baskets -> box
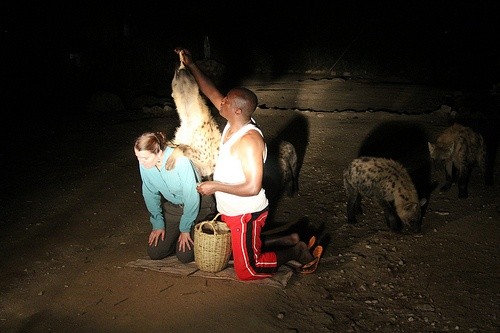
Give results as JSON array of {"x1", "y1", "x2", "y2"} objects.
[{"x1": 193, "y1": 212, "x2": 231, "y2": 272}]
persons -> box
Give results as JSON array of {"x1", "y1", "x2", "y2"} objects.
[
  {"x1": 175, "y1": 47, "x2": 323, "y2": 281},
  {"x1": 133, "y1": 131, "x2": 217, "y2": 263}
]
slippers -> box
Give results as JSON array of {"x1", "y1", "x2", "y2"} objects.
[
  {"x1": 297, "y1": 245, "x2": 323, "y2": 273},
  {"x1": 307, "y1": 236, "x2": 317, "y2": 250}
]
